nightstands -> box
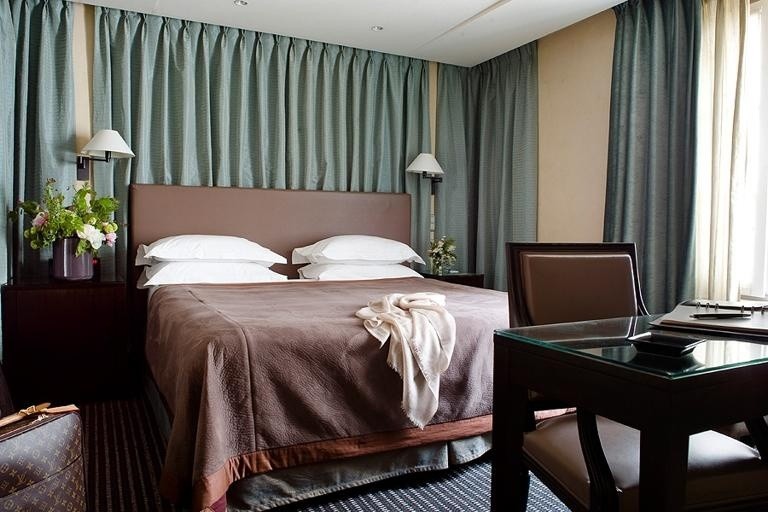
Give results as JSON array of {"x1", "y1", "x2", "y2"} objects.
[
  {"x1": 418, "y1": 270, "x2": 485, "y2": 288},
  {"x1": 0, "y1": 271, "x2": 127, "y2": 399}
]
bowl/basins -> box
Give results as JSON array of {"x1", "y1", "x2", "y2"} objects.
[
  {"x1": 622, "y1": 330, "x2": 707, "y2": 358},
  {"x1": 626, "y1": 351, "x2": 703, "y2": 377}
]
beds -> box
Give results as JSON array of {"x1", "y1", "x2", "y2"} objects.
[{"x1": 125, "y1": 181, "x2": 581, "y2": 512}]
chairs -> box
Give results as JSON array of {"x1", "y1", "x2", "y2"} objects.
[{"x1": 505, "y1": 240, "x2": 768, "y2": 511}]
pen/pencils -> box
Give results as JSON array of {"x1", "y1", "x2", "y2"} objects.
[{"x1": 690, "y1": 313, "x2": 751, "y2": 319}]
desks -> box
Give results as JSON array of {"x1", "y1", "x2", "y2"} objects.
[{"x1": 489, "y1": 311, "x2": 768, "y2": 512}]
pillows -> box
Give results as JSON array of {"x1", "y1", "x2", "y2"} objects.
[
  {"x1": 291, "y1": 233, "x2": 426, "y2": 266},
  {"x1": 295, "y1": 263, "x2": 424, "y2": 281},
  {"x1": 136, "y1": 262, "x2": 289, "y2": 290},
  {"x1": 133, "y1": 233, "x2": 289, "y2": 269}
]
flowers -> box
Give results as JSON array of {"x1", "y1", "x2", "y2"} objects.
[
  {"x1": 15, "y1": 176, "x2": 124, "y2": 255},
  {"x1": 427, "y1": 235, "x2": 458, "y2": 269}
]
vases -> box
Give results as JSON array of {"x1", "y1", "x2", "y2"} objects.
[{"x1": 52, "y1": 234, "x2": 96, "y2": 282}]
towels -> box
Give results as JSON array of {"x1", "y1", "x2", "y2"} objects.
[{"x1": 354, "y1": 291, "x2": 458, "y2": 431}]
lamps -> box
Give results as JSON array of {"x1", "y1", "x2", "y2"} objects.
[
  {"x1": 405, "y1": 152, "x2": 446, "y2": 195},
  {"x1": 75, "y1": 128, "x2": 137, "y2": 181}
]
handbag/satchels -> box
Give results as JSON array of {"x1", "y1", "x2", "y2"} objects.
[{"x1": 0, "y1": 402, "x2": 89, "y2": 512}]
